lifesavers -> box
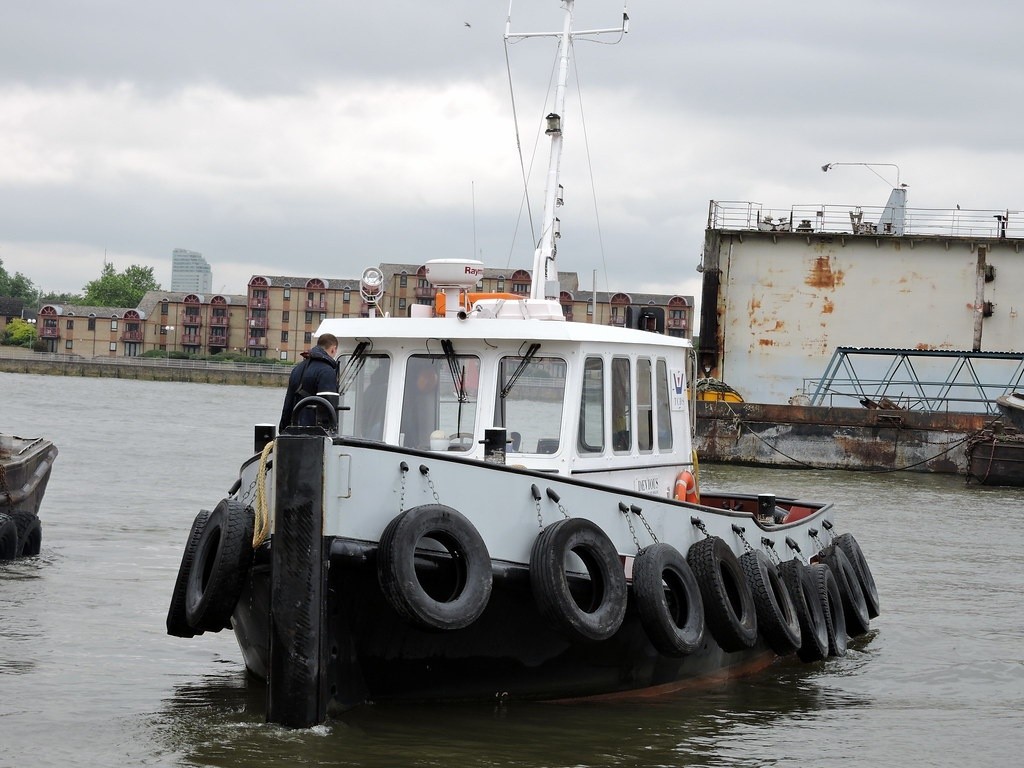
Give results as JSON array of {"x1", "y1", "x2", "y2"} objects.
[
  {"x1": 184, "y1": 498, "x2": 256, "y2": 633},
  {"x1": 166, "y1": 509, "x2": 213, "y2": 638},
  {"x1": 835, "y1": 534, "x2": 879, "y2": 619},
  {"x1": 776, "y1": 559, "x2": 829, "y2": 664},
  {"x1": 632, "y1": 545, "x2": 705, "y2": 658},
  {"x1": 11, "y1": 511, "x2": 42, "y2": 558},
  {"x1": 674, "y1": 472, "x2": 698, "y2": 505},
  {"x1": 530, "y1": 518, "x2": 628, "y2": 644},
  {"x1": 687, "y1": 536, "x2": 758, "y2": 654},
  {"x1": 0, "y1": 512, "x2": 18, "y2": 563},
  {"x1": 818, "y1": 547, "x2": 870, "y2": 638},
  {"x1": 733, "y1": 552, "x2": 803, "y2": 655},
  {"x1": 806, "y1": 563, "x2": 848, "y2": 658},
  {"x1": 376, "y1": 503, "x2": 493, "y2": 634}
]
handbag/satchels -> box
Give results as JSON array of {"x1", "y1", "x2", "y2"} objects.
[{"x1": 294, "y1": 357, "x2": 333, "y2": 398}]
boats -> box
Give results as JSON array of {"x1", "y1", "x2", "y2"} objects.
[
  {"x1": 0, "y1": 436, "x2": 59, "y2": 564},
  {"x1": 161, "y1": 1, "x2": 880, "y2": 733}
]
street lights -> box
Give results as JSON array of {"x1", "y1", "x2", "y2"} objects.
[
  {"x1": 165, "y1": 325, "x2": 175, "y2": 367},
  {"x1": 28, "y1": 318, "x2": 37, "y2": 351}
]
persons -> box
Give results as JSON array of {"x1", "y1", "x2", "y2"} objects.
[{"x1": 278, "y1": 333, "x2": 339, "y2": 437}]
